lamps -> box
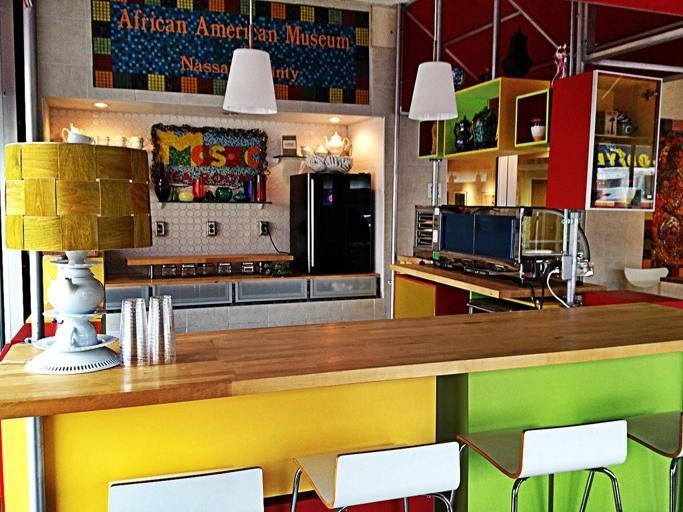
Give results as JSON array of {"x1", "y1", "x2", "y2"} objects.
[
  {"x1": 222, "y1": 0, "x2": 278, "y2": 114},
  {"x1": 4, "y1": 142, "x2": 153, "y2": 375},
  {"x1": 408, "y1": 0, "x2": 459, "y2": 121}
]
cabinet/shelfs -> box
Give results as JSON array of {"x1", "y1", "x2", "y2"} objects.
[
  {"x1": 443, "y1": 78, "x2": 552, "y2": 160},
  {"x1": 514, "y1": 88, "x2": 550, "y2": 148},
  {"x1": 546, "y1": 69, "x2": 663, "y2": 212},
  {"x1": 417, "y1": 120, "x2": 444, "y2": 160}
]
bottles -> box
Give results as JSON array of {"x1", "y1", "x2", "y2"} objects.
[{"x1": 155, "y1": 177, "x2": 232, "y2": 202}]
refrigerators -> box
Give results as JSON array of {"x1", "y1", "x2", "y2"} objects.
[{"x1": 289, "y1": 173, "x2": 374, "y2": 276}]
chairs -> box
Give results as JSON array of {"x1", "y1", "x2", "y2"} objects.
[{"x1": 624, "y1": 266, "x2": 668, "y2": 295}]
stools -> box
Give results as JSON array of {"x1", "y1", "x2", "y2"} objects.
[
  {"x1": 290, "y1": 442, "x2": 463, "y2": 512},
  {"x1": 445, "y1": 416, "x2": 628, "y2": 512},
  {"x1": 107, "y1": 463, "x2": 264, "y2": 511},
  {"x1": 580, "y1": 414, "x2": 683, "y2": 510}
]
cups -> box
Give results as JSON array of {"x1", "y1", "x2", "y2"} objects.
[
  {"x1": 62, "y1": 128, "x2": 93, "y2": 145},
  {"x1": 531, "y1": 121, "x2": 545, "y2": 142},
  {"x1": 55, "y1": 320, "x2": 100, "y2": 349},
  {"x1": 244, "y1": 174, "x2": 266, "y2": 202},
  {"x1": 119, "y1": 295, "x2": 176, "y2": 366}
]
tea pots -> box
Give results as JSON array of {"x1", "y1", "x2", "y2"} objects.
[
  {"x1": 454, "y1": 115, "x2": 474, "y2": 152},
  {"x1": 324, "y1": 130, "x2": 350, "y2": 155},
  {"x1": 50, "y1": 268, "x2": 105, "y2": 314}
]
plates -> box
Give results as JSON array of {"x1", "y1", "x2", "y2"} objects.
[
  {"x1": 43, "y1": 311, "x2": 105, "y2": 320},
  {"x1": 30, "y1": 332, "x2": 116, "y2": 350},
  {"x1": 47, "y1": 261, "x2": 100, "y2": 267}
]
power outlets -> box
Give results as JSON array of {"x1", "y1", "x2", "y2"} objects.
[
  {"x1": 260, "y1": 221, "x2": 269, "y2": 236},
  {"x1": 155, "y1": 222, "x2": 165, "y2": 235},
  {"x1": 207, "y1": 221, "x2": 216, "y2": 235}
]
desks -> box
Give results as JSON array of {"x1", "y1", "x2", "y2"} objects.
[
  {"x1": 1, "y1": 303, "x2": 682, "y2": 512},
  {"x1": 390, "y1": 264, "x2": 607, "y2": 319}
]
